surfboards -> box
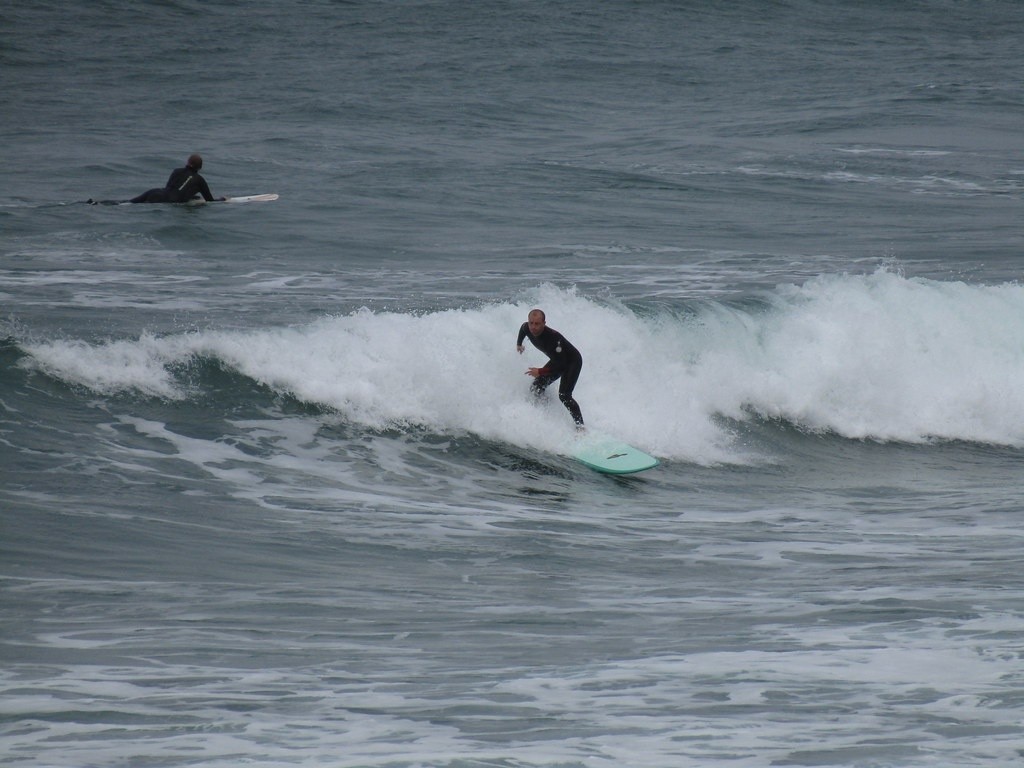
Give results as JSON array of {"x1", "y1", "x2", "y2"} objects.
[
  {"x1": 76, "y1": 192, "x2": 279, "y2": 205},
  {"x1": 554, "y1": 420, "x2": 662, "y2": 476}
]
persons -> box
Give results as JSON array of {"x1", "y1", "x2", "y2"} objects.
[
  {"x1": 517, "y1": 309, "x2": 585, "y2": 432},
  {"x1": 86, "y1": 154, "x2": 228, "y2": 205}
]
